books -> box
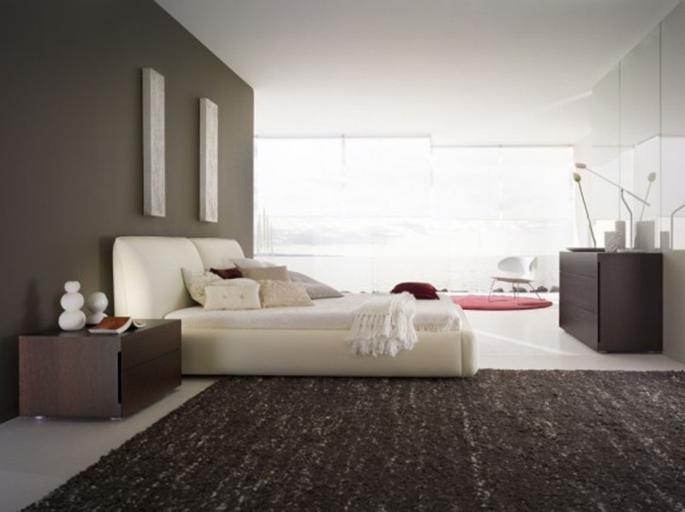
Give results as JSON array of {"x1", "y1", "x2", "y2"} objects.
[{"x1": 88, "y1": 315, "x2": 146, "y2": 335}]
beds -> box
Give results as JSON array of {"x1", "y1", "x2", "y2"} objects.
[{"x1": 110, "y1": 234, "x2": 484, "y2": 382}]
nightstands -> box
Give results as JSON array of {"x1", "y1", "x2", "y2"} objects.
[{"x1": 15, "y1": 316, "x2": 185, "y2": 429}]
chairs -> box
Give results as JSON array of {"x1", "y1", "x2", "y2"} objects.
[{"x1": 488, "y1": 252, "x2": 545, "y2": 306}]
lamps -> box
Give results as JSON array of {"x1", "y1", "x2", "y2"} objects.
[{"x1": 573, "y1": 160, "x2": 652, "y2": 250}]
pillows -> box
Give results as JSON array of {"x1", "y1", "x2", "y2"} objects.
[
  {"x1": 180, "y1": 253, "x2": 347, "y2": 312},
  {"x1": 389, "y1": 281, "x2": 440, "y2": 304}
]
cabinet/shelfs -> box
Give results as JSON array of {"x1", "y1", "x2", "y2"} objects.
[{"x1": 556, "y1": 246, "x2": 666, "y2": 360}]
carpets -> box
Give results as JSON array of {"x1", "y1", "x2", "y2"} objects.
[
  {"x1": 16, "y1": 370, "x2": 684, "y2": 510},
  {"x1": 450, "y1": 290, "x2": 556, "y2": 315}
]
